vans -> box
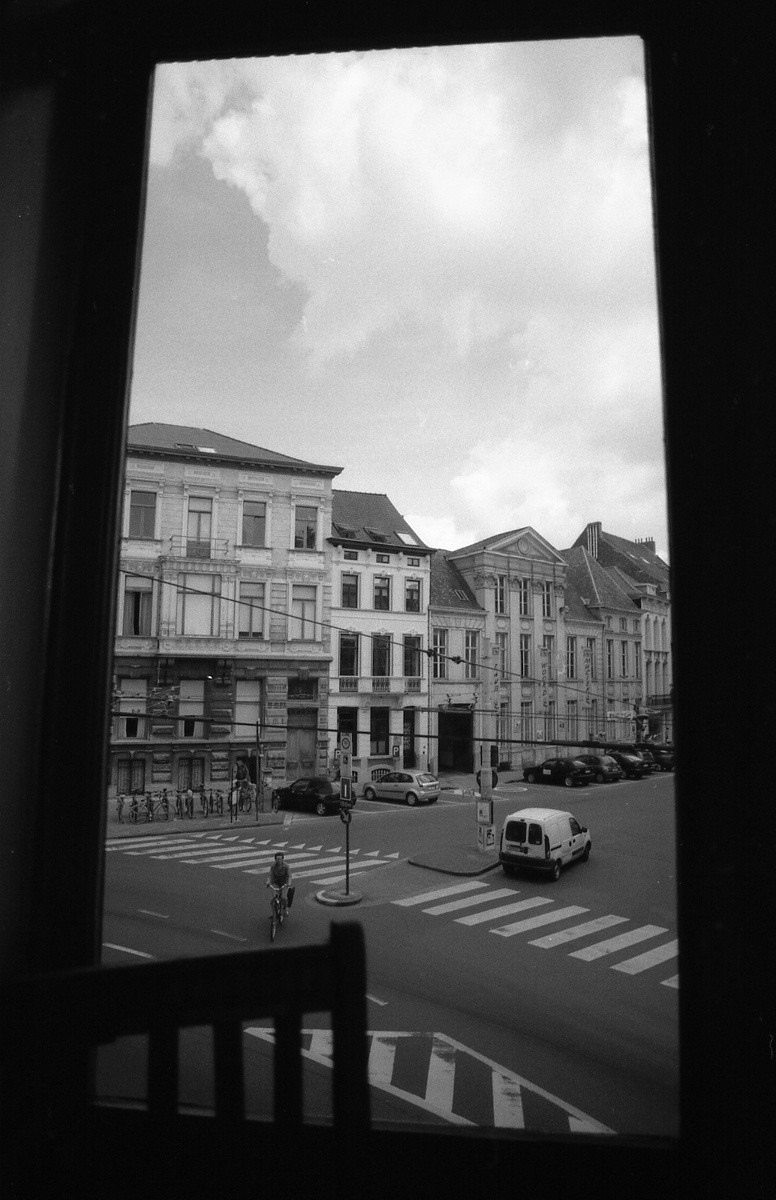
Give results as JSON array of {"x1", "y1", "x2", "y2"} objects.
[{"x1": 498, "y1": 807, "x2": 592, "y2": 883}]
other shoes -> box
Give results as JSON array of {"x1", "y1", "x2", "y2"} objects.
[
  {"x1": 269, "y1": 914, "x2": 273, "y2": 919},
  {"x1": 284, "y1": 909, "x2": 289, "y2": 917}
]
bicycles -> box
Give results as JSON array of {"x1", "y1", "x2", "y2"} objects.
[
  {"x1": 115, "y1": 777, "x2": 254, "y2": 826},
  {"x1": 263, "y1": 882, "x2": 295, "y2": 943}
]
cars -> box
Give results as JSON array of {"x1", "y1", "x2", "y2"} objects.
[
  {"x1": 605, "y1": 750, "x2": 652, "y2": 780},
  {"x1": 627, "y1": 747, "x2": 675, "y2": 773},
  {"x1": 271, "y1": 774, "x2": 357, "y2": 816},
  {"x1": 522, "y1": 757, "x2": 596, "y2": 788},
  {"x1": 574, "y1": 753, "x2": 625, "y2": 784},
  {"x1": 361, "y1": 768, "x2": 442, "y2": 806}
]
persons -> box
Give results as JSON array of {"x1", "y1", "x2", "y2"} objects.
[
  {"x1": 232, "y1": 759, "x2": 250, "y2": 805},
  {"x1": 265, "y1": 853, "x2": 292, "y2": 918}
]
